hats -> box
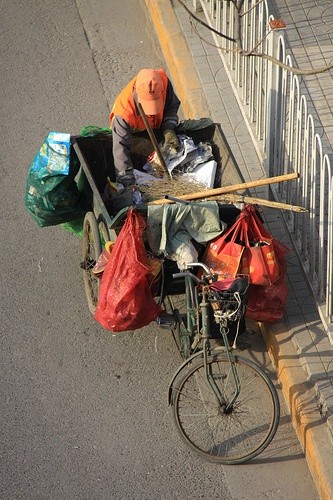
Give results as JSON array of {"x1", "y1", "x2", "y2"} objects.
[{"x1": 136, "y1": 69, "x2": 164, "y2": 115}]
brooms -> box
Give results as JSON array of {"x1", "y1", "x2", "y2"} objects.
[{"x1": 132, "y1": 164, "x2": 308, "y2": 212}]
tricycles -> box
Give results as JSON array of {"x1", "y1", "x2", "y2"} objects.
[{"x1": 70, "y1": 122, "x2": 282, "y2": 466}]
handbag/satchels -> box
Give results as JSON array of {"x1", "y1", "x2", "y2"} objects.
[{"x1": 196, "y1": 205, "x2": 283, "y2": 291}]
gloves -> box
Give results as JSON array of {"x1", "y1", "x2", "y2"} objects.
[{"x1": 163, "y1": 129, "x2": 180, "y2": 149}]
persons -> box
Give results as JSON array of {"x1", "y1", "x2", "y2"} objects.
[{"x1": 108, "y1": 68, "x2": 181, "y2": 188}]
